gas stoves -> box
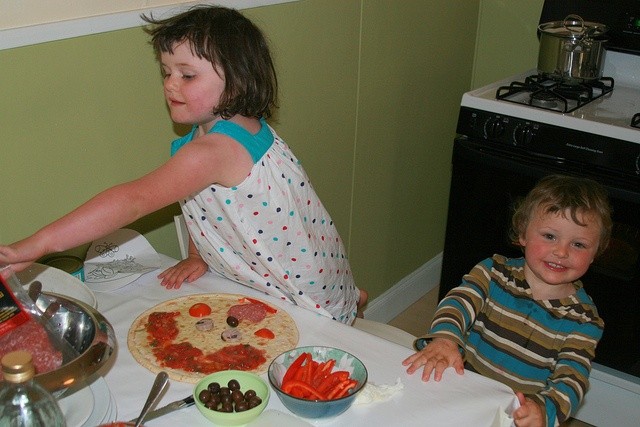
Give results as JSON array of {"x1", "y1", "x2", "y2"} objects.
[{"x1": 496, "y1": 71, "x2": 613, "y2": 114}]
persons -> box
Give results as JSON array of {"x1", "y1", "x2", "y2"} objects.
[
  {"x1": 0, "y1": 2, "x2": 360, "y2": 332},
  {"x1": 401, "y1": 171, "x2": 615, "y2": 427}
]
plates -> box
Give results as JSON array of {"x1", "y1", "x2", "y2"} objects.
[
  {"x1": 54, "y1": 374, "x2": 118, "y2": 427},
  {"x1": 16, "y1": 262, "x2": 97, "y2": 311}
]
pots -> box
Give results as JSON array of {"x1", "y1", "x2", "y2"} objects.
[{"x1": 537, "y1": 13, "x2": 610, "y2": 79}]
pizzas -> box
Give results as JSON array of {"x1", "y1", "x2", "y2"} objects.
[{"x1": 127, "y1": 292, "x2": 299, "y2": 384}]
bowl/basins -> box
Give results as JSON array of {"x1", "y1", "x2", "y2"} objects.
[
  {"x1": 268, "y1": 346, "x2": 369, "y2": 420},
  {"x1": 2, "y1": 289, "x2": 118, "y2": 405},
  {"x1": 196, "y1": 369, "x2": 269, "y2": 427}
]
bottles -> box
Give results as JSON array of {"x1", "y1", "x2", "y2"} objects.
[{"x1": 0, "y1": 350, "x2": 68, "y2": 427}]
